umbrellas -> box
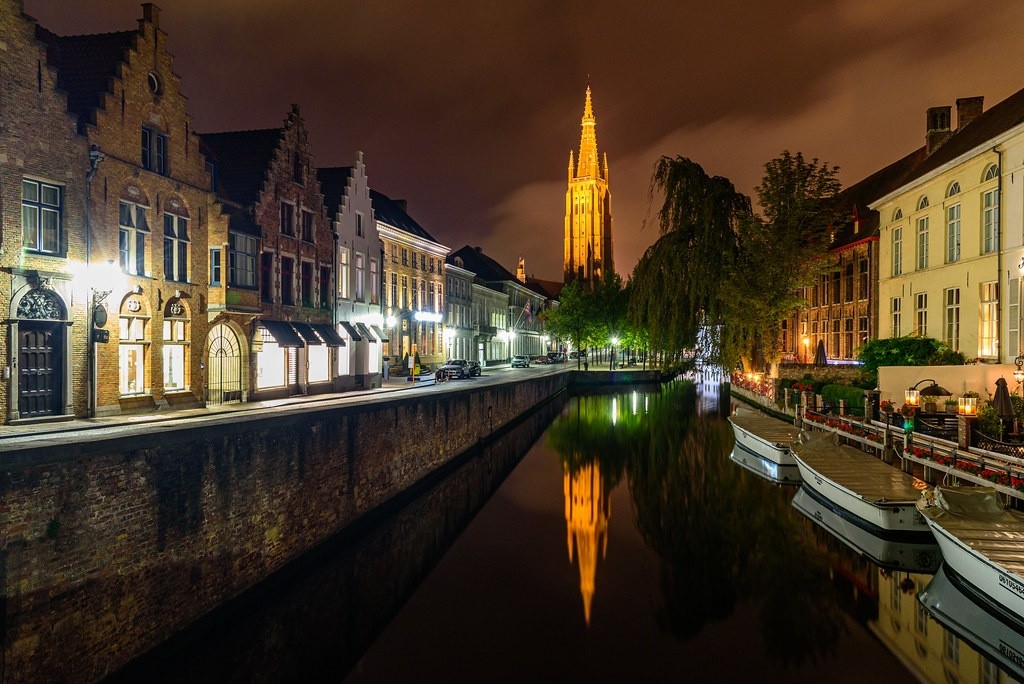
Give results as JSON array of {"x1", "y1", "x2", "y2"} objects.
[
  {"x1": 814, "y1": 339, "x2": 827, "y2": 364},
  {"x1": 993, "y1": 377, "x2": 1015, "y2": 442}
]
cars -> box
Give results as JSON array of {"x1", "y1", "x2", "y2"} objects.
[
  {"x1": 571, "y1": 352, "x2": 578, "y2": 359},
  {"x1": 535, "y1": 356, "x2": 553, "y2": 365}
]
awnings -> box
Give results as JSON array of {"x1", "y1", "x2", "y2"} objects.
[
  {"x1": 371, "y1": 325, "x2": 389, "y2": 343},
  {"x1": 259, "y1": 320, "x2": 304, "y2": 348},
  {"x1": 340, "y1": 320, "x2": 362, "y2": 342},
  {"x1": 310, "y1": 322, "x2": 346, "y2": 348},
  {"x1": 290, "y1": 321, "x2": 322, "y2": 345},
  {"x1": 356, "y1": 322, "x2": 377, "y2": 343}
]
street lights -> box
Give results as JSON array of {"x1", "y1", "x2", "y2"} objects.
[
  {"x1": 804, "y1": 338, "x2": 808, "y2": 362},
  {"x1": 613, "y1": 338, "x2": 618, "y2": 360}
]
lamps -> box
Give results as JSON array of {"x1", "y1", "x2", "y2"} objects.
[
  {"x1": 959, "y1": 394, "x2": 978, "y2": 417},
  {"x1": 1013, "y1": 356, "x2": 1024, "y2": 384},
  {"x1": 905, "y1": 386, "x2": 920, "y2": 406}
]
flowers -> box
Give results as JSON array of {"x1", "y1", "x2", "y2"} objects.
[
  {"x1": 902, "y1": 404, "x2": 915, "y2": 419},
  {"x1": 880, "y1": 399, "x2": 896, "y2": 414},
  {"x1": 904, "y1": 446, "x2": 1024, "y2": 494},
  {"x1": 804, "y1": 412, "x2": 884, "y2": 444}
]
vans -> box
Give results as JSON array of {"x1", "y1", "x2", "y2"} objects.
[{"x1": 547, "y1": 353, "x2": 564, "y2": 363}]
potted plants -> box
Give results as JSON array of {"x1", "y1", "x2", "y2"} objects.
[
  {"x1": 944, "y1": 400, "x2": 958, "y2": 414},
  {"x1": 920, "y1": 395, "x2": 940, "y2": 413}
]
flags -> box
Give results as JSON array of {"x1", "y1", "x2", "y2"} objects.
[{"x1": 523, "y1": 299, "x2": 542, "y2": 324}]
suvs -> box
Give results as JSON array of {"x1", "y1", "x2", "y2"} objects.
[
  {"x1": 442, "y1": 359, "x2": 471, "y2": 378},
  {"x1": 468, "y1": 361, "x2": 482, "y2": 376},
  {"x1": 511, "y1": 355, "x2": 530, "y2": 368}
]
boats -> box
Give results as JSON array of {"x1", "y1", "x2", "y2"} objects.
[
  {"x1": 728, "y1": 441, "x2": 801, "y2": 485},
  {"x1": 918, "y1": 560, "x2": 1024, "y2": 684},
  {"x1": 789, "y1": 429, "x2": 932, "y2": 533},
  {"x1": 727, "y1": 404, "x2": 840, "y2": 464},
  {"x1": 916, "y1": 482, "x2": 1024, "y2": 619},
  {"x1": 790, "y1": 481, "x2": 942, "y2": 575}
]
